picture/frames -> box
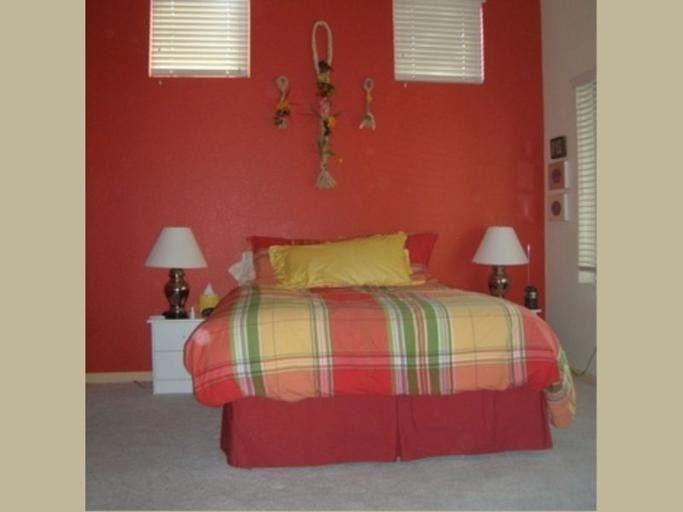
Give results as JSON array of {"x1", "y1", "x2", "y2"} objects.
[{"x1": 550, "y1": 137, "x2": 566, "y2": 159}]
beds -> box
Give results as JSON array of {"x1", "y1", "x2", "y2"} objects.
[{"x1": 181, "y1": 233, "x2": 578, "y2": 468}]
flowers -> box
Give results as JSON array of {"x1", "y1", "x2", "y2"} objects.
[
  {"x1": 275, "y1": 99, "x2": 289, "y2": 114},
  {"x1": 323, "y1": 112, "x2": 336, "y2": 135}
]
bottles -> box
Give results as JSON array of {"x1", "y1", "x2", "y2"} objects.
[{"x1": 525, "y1": 286, "x2": 538, "y2": 310}]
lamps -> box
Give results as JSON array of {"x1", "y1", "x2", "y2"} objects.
[
  {"x1": 471, "y1": 226, "x2": 530, "y2": 299},
  {"x1": 144, "y1": 227, "x2": 208, "y2": 319}
]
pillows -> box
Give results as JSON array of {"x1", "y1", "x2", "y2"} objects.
[
  {"x1": 228, "y1": 249, "x2": 255, "y2": 288},
  {"x1": 242, "y1": 232, "x2": 440, "y2": 291},
  {"x1": 268, "y1": 230, "x2": 414, "y2": 291}
]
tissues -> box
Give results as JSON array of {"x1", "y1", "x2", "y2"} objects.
[{"x1": 199, "y1": 282, "x2": 219, "y2": 310}]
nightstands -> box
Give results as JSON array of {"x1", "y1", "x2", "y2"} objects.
[
  {"x1": 527, "y1": 307, "x2": 542, "y2": 317},
  {"x1": 147, "y1": 314, "x2": 207, "y2": 395}
]
